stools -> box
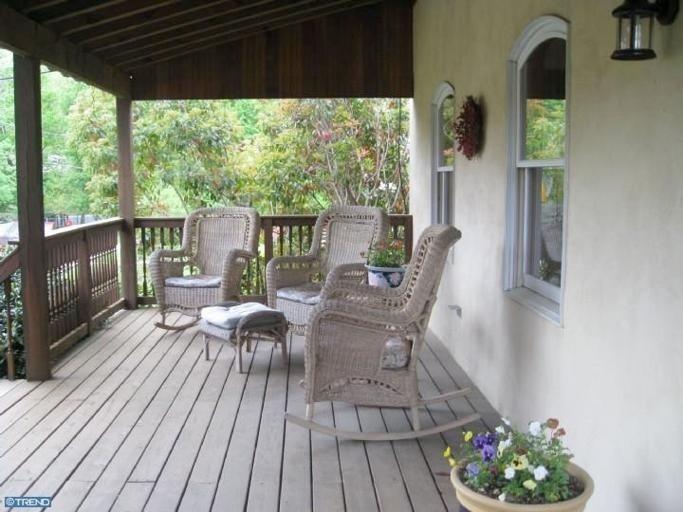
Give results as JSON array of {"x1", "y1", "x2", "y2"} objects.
[{"x1": 201, "y1": 304, "x2": 288, "y2": 374}]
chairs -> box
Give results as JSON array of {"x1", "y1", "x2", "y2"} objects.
[
  {"x1": 265, "y1": 204, "x2": 389, "y2": 349},
  {"x1": 150, "y1": 207, "x2": 261, "y2": 331},
  {"x1": 282, "y1": 224, "x2": 480, "y2": 442}
]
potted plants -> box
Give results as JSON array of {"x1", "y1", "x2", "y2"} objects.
[{"x1": 360, "y1": 238, "x2": 406, "y2": 288}]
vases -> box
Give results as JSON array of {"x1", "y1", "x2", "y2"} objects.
[{"x1": 449, "y1": 452, "x2": 594, "y2": 512}]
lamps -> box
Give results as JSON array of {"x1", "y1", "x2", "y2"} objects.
[{"x1": 610, "y1": 1, "x2": 683, "y2": 60}]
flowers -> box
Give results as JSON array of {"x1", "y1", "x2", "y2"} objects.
[{"x1": 441, "y1": 417, "x2": 573, "y2": 502}]
